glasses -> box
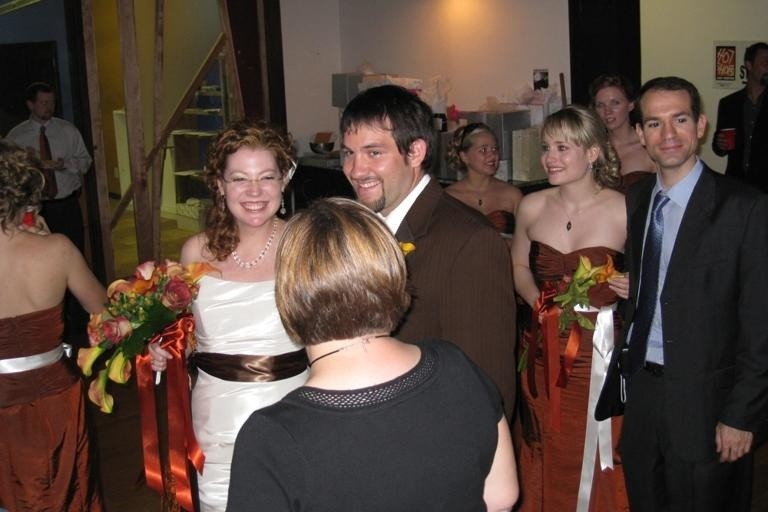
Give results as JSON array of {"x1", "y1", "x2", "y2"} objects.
[{"x1": 221, "y1": 172, "x2": 283, "y2": 187}]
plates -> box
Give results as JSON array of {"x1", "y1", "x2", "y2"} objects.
[{"x1": 40, "y1": 167, "x2": 54, "y2": 169}]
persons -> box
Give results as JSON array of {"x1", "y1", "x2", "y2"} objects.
[
  {"x1": 149, "y1": 120, "x2": 313, "y2": 512},
  {"x1": 0, "y1": 137, "x2": 109, "y2": 512},
  {"x1": 712, "y1": 43, "x2": 766, "y2": 193},
  {"x1": 341, "y1": 85, "x2": 517, "y2": 428},
  {"x1": 595, "y1": 76, "x2": 768, "y2": 512},
  {"x1": 226, "y1": 197, "x2": 521, "y2": 512},
  {"x1": 511, "y1": 105, "x2": 630, "y2": 512},
  {"x1": 5, "y1": 82, "x2": 93, "y2": 328}
]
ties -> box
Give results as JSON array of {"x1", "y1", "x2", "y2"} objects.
[
  {"x1": 627, "y1": 190, "x2": 670, "y2": 381},
  {"x1": 40, "y1": 126, "x2": 57, "y2": 198}
]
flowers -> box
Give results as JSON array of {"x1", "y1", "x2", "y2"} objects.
[
  {"x1": 76, "y1": 258, "x2": 222, "y2": 413},
  {"x1": 555, "y1": 250, "x2": 629, "y2": 346}
]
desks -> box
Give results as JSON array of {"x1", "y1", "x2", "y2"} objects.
[{"x1": 292, "y1": 146, "x2": 550, "y2": 215}]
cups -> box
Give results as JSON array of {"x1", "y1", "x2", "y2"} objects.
[
  {"x1": 721, "y1": 129, "x2": 735, "y2": 149},
  {"x1": 41, "y1": 160, "x2": 56, "y2": 167},
  {"x1": 23, "y1": 205, "x2": 34, "y2": 226}
]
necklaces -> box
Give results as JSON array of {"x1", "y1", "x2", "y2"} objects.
[{"x1": 309, "y1": 335, "x2": 393, "y2": 366}]
